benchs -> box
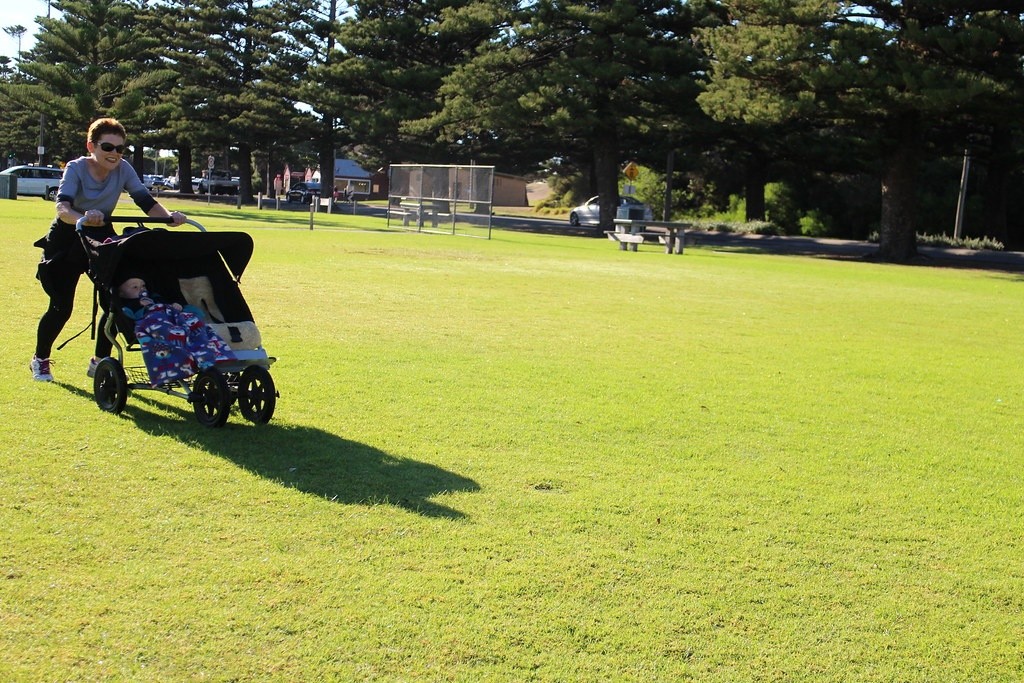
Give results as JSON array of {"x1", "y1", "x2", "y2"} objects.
[
  {"x1": 387, "y1": 210, "x2": 412, "y2": 217},
  {"x1": 629, "y1": 231, "x2": 688, "y2": 239},
  {"x1": 427, "y1": 213, "x2": 453, "y2": 218},
  {"x1": 603, "y1": 230, "x2": 665, "y2": 236}
]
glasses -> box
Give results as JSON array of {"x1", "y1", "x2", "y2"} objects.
[{"x1": 96, "y1": 140, "x2": 127, "y2": 154}]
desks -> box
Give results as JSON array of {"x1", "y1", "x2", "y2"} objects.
[
  {"x1": 399, "y1": 202, "x2": 441, "y2": 228},
  {"x1": 608, "y1": 218, "x2": 693, "y2": 255}
]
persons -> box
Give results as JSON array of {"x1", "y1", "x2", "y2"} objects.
[
  {"x1": 111, "y1": 265, "x2": 203, "y2": 352},
  {"x1": 30, "y1": 118, "x2": 187, "y2": 382}
]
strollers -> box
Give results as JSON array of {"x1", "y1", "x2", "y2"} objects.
[{"x1": 74, "y1": 212, "x2": 281, "y2": 428}]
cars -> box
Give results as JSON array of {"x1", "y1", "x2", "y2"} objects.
[
  {"x1": 285, "y1": 180, "x2": 329, "y2": 205},
  {"x1": 570, "y1": 194, "x2": 654, "y2": 229},
  {"x1": 143, "y1": 175, "x2": 242, "y2": 190}
]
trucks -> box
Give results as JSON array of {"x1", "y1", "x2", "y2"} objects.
[{"x1": 198, "y1": 169, "x2": 239, "y2": 196}]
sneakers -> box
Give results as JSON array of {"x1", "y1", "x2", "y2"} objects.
[
  {"x1": 30, "y1": 353, "x2": 54, "y2": 382},
  {"x1": 87, "y1": 356, "x2": 130, "y2": 381}
]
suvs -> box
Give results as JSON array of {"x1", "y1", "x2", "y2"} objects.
[{"x1": 0, "y1": 165, "x2": 64, "y2": 202}]
